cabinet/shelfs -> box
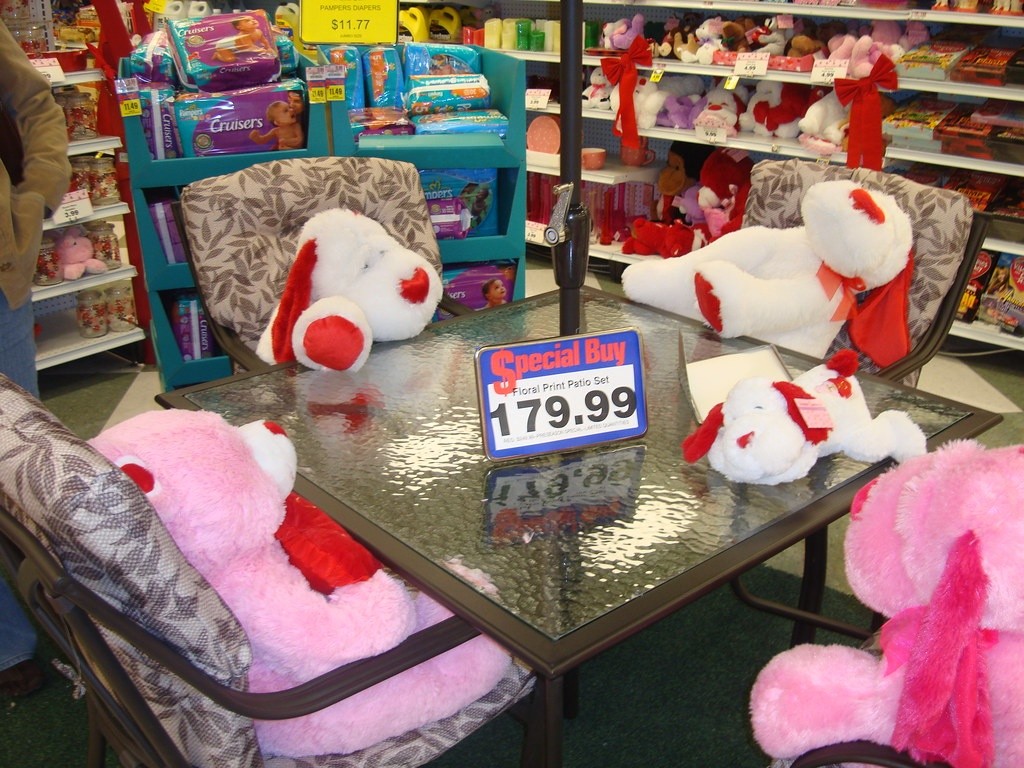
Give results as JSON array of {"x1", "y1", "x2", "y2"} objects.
[{"x1": 0, "y1": 0, "x2": 1024, "y2": 373}]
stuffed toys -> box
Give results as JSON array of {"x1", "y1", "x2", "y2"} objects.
[
  {"x1": 80, "y1": 409, "x2": 511, "y2": 756},
  {"x1": 581, "y1": 68, "x2": 896, "y2": 154},
  {"x1": 256, "y1": 207, "x2": 445, "y2": 375},
  {"x1": 749, "y1": 437, "x2": 1023, "y2": 768},
  {"x1": 620, "y1": 180, "x2": 914, "y2": 367},
  {"x1": 623, "y1": 140, "x2": 753, "y2": 260},
  {"x1": 681, "y1": 352, "x2": 927, "y2": 484},
  {"x1": 598, "y1": 12, "x2": 929, "y2": 79},
  {"x1": 45, "y1": 227, "x2": 107, "y2": 279},
  {"x1": 475, "y1": 2, "x2": 501, "y2": 21}
]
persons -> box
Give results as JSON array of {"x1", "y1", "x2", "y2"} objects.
[
  {"x1": 249, "y1": 91, "x2": 307, "y2": 150},
  {"x1": 482, "y1": 279, "x2": 507, "y2": 308},
  {"x1": 211, "y1": 17, "x2": 273, "y2": 62},
  {"x1": 0, "y1": 17, "x2": 72, "y2": 699},
  {"x1": 461, "y1": 183, "x2": 488, "y2": 223}
]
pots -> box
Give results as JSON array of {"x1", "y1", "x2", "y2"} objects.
[{"x1": 42, "y1": 46, "x2": 89, "y2": 72}]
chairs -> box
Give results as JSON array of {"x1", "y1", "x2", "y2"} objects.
[
  {"x1": 731, "y1": 158, "x2": 993, "y2": 647},
  {"x1": 0, "y1": 373, "x2": 542, "y2": 768},
  {"x1": 171, "y1": 156, "x2": 474, "y2": 372}
]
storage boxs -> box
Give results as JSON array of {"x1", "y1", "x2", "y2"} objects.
[
  {"x1": 895, "y1": 27, "x2": 991, "y2": 81},
  {"x1": 949, "y1": 36, "x2": 1024, "y2": 86},
  {"x1": 882, "y1": 95, "x2": 959, "y2": 138},
  {"x1": 933, "y1": 104, "x2": 999, "y2": 146}
]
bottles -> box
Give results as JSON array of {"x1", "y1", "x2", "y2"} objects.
[{"x1": 2, "y1": 8, "x2": 137, "y2": 338}]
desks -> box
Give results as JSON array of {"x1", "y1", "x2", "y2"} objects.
[{"x1": 153, "y1": 286, "x2": 1005, "y2": 768}]
